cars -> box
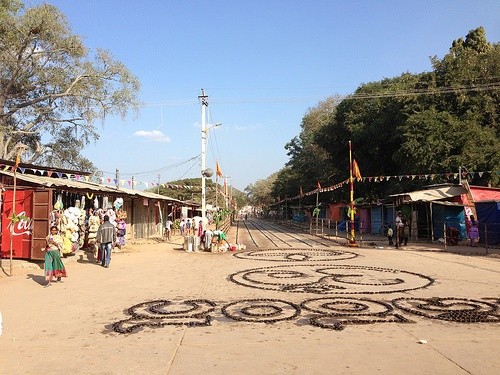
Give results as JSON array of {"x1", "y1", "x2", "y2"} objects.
[{"x1": 207, "y1": 217, "x2": 212, "y2": 225}]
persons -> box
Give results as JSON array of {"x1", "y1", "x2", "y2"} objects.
[
  {"x1": 97, "y1": 219, "x2": 107, "y2": 265},
  {"x1": 205, "y1": 230, "x2": 213, "y2": 249},
  {"x1": 200, "y1": 230, "x2": 207, "y2": 249},
  {"x1": 41, "y1": 226, "x2": 67, "y2": 288},
  {"x1": 179, "y1": 219, "x2": 186, "y2": 235},
  {"x1": 235, "y1": 206, "x2": 281, "y2": 222},
  {"x1": 198, "y1": 220, "x2": 204, "y2": 237},
  {"x1": 387, "y1": 210, "x2": 411, "y2": 248},
  {"x1": 192, "y1": 219, "x2": 196, "y2": 235},
  {"x1": 165, "y1": 218, "x2": 173, "y2": 239},
  {"x1": 97, "y1": 215, "x2": 116, "y2": 268},
  {"x1": 78, "y1": 207, "x2": 123, "y2": 253},
  {"x1": 305, "y1": 207, "x2": 312, "y2": 226},
  {"x1": 467, "y1": 215, "x2": 479, "y2": 246},
  {"x1": 186, "y1": 219, "x2": 191, "y2": 236},
  {"x1": 212, "y1": 229, "x2": 226, "y2": 244}
]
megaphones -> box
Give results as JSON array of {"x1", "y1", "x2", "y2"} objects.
[{"x1": 201, "y1": 168, "x2": 213, "y2": 177}]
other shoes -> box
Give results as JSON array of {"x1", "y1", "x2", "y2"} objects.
[
  {"x1": 404, "y1": 243, "x2": 407, "y2": 246},
  {"x1": 55, "y1": 277, "x2": 61, "y2": 282},
  {"x1": 389, "y1": 242, "x2": 392, "y2": 245},
  {"x1": 45, "y1": 283, "x2": 52, "y2": 287},
  {"x1": 100, "y1": 263, "x2": 108, "y2": 268}
]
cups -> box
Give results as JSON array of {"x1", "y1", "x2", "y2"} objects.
[{"x1": 49, "y1": 236, "x2": 53, "y2": 241}]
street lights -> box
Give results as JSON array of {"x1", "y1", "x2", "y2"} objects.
[{"x1": 201, "y1": 122, "x2": 223, "y2": 229}]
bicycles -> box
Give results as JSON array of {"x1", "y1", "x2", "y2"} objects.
[{"x1": 164, "y1": 229, "x2": 171, "y2": 241}]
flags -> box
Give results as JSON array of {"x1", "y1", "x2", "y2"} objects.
[
  {"x1": 216, "y1": 161, "x2": 222, "y2": 178},
  {"x1": 352, "y1": 160, "x2": 362, "y2": 182},
  {"x1": 318, "y1": 182, "x2": 322, "y2": 189}
]
